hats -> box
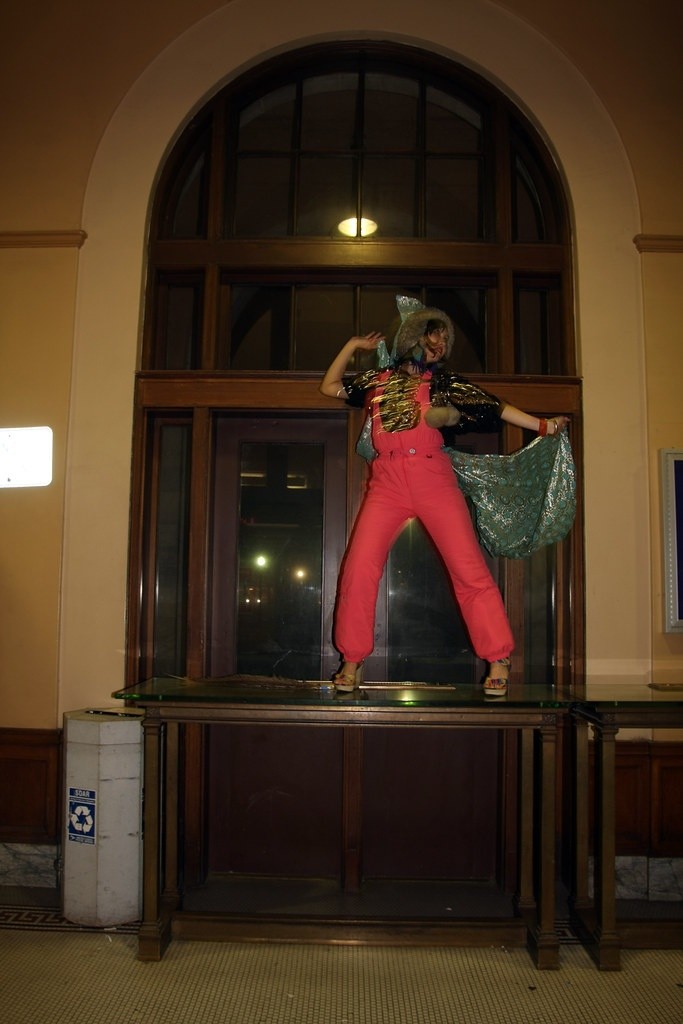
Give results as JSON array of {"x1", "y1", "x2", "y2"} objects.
[{"x1": 397, "y1": 307, "x2": 454, "y2": 362}]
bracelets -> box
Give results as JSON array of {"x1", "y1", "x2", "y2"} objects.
[
  {"x1": 537, "y1": 417, "x2": 548, "y2": 437},
  {"x1": 550, "y1": 417, "x2": 558, "y2": 436}
]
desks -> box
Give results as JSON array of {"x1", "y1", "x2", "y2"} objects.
[{"x1": 107, "y1": 678, "x2": 683, "y2": 972}]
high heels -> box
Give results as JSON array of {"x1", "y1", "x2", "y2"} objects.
[
  {"x1": 483, "y1": 658, "x2": 512, "y2": 695},
  {"x1": 332, "y1": 662, "x2": 363, "y2": 691}
]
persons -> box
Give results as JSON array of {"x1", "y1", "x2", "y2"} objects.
[{"x1": 318, "y1": 294, "x2": 570, "y2": 699}]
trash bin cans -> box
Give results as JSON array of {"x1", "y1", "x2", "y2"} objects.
[{"x1": 58, "y1": 706, "x2": 144, "y2": 927}]
